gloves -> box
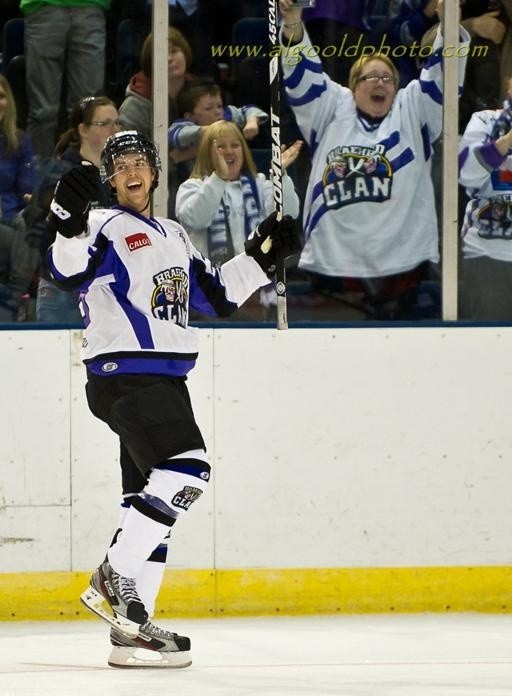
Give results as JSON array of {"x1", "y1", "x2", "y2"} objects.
[
  {"x1": 47, "y1": 165, "x2": 105, "y2": 239},
  {"x1": 244, "y1": 210, "x2": 301, "y2": 278}
]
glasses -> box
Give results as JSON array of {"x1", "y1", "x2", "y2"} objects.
[
  {"x1": 357, "y1": 72, "x2": 396, "y2": 84},
  {"x1": 87, "y1": 120, "x2": 121, "y2": 132}
]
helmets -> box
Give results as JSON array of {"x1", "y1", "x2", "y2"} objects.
[{"x1": 100, "y1": 128, "x2": 162, "y2": 193}]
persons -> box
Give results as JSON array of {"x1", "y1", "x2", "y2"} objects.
[
  {"x1": 1, "y1": 0, "x2": 509, "y2": 322},
  {"x1": 39, "y1": 129, "x2": 303, "y2": 658}
]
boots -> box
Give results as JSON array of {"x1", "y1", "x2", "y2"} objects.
[{"x1": 90, "y1": 553, "x2": 190, "y2": 651}]
